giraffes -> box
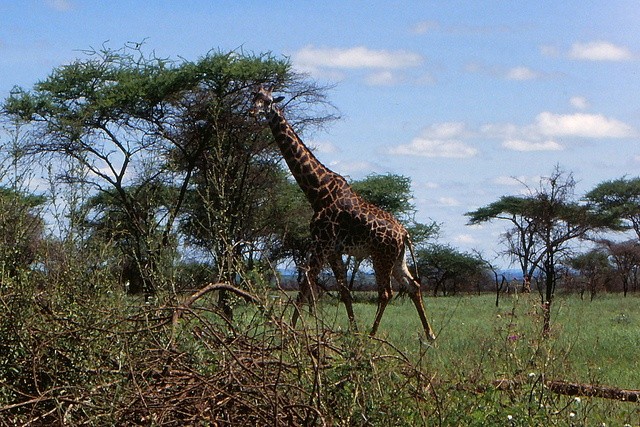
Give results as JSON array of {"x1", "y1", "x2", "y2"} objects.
[{"x1": 247, "y1": 82, "x2": 437, "y2": 343}]
